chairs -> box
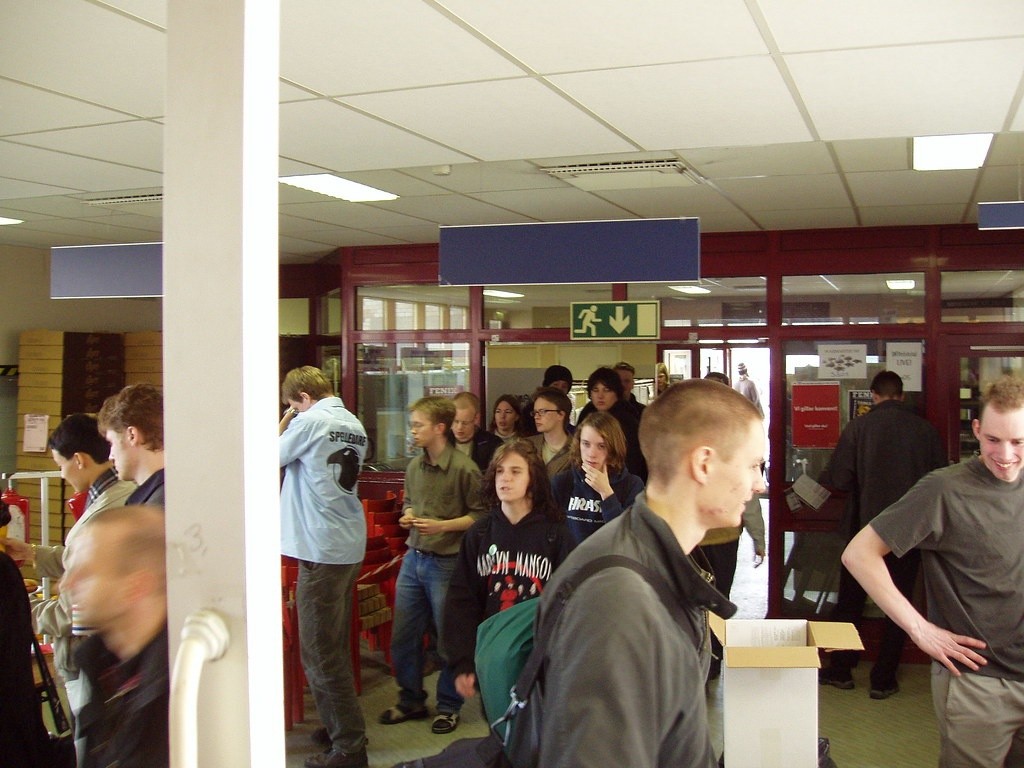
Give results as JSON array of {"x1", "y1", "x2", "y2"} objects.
[{"x1": 279, "y1": 488, "x2": 429, "y2": 732}]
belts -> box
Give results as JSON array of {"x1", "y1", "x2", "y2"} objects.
[{"x1": 415, "y1": 548, "x2": 456, "y2": 558}]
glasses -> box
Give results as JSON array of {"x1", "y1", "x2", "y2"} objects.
[{"x1": 530, "y1": 408, "x2": 560, "y2": 417}]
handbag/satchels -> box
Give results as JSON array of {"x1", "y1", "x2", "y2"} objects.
[
  {"x1": 391, "y1": 736, "x2": 511, "y2": 768},
  {"x1": 474, "y1": 597, "x2": 542, "y2": 760}
]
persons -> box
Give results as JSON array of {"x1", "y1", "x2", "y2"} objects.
[
  {"x1": 281, "y1": 366, "x2": 372, "y2": 767},
  {"x1": 506, "y1": 378, "x2": 770, "y2": 768},
  {"x1": 376, "y1": 361, "x2": 765, "y2": 736},
  {"x1": 840, "y1": 373, "x2": 1024, "y2": 768},
  {"x1": 819, "y1": 371, "x2": 950, "y2": 699},
  {"x1": 0, "y1": 382, "x2": 172, "y2": 768}
]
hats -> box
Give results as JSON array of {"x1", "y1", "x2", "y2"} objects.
[
  {"x1": 737, "y1": 363, "x2": 747, "y2": 374},
  {"x1": 542, "y1": 365, "x2": 572, "y2": 394},
  {"x1": 613, "y1": 362, "x2": 635, "y2": 375}
]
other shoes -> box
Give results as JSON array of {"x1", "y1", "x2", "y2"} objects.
[
  {"x1": 819, "y1": 668, "x2": 855, "y2": 689},
  {"x1": 870, "y1": 680, "x2": 899, "y2": 699},
  {"x1": 303, "y1": 749, "x2": 367, "y2": 768},
  {"x1": 379, "y1": 703, "x2": 428, "y2": 724},
  {"x1": 432, "y1": 712, "x2": 459, "y2": 733},
  {"x1": 310, "y1": 727, "x2": 333, "y2": 745}
]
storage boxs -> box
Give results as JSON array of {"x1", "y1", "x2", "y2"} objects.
[{"x1": 705, "y1": 611, "x2": 866, "y2": 768}]
